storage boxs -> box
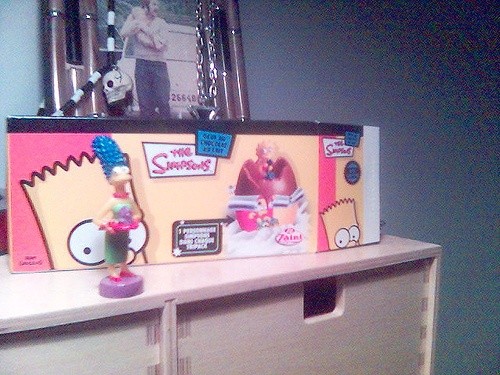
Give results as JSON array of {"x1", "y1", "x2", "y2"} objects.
[{"x1": 4, "y1": 113, "x2": 385, "y2": 276}]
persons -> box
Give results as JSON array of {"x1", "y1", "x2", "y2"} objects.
[
  {"x1": 119, "y1": 0, "x2": 173, "y2": 120},
  {"x1": 132, "y1": 6, "x2": 167, "y2": 50}
]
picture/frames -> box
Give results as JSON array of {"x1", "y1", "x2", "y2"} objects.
[{"x1": 45, "y1": 2, "x2": 251, "y2": 124}]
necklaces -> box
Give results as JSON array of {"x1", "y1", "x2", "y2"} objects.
[{"x1": 187, "y1": 0, "x2": 222, "y2": 120}]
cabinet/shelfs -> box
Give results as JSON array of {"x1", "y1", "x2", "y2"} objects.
[{"x1": 2, "y1": 229, "x2": 441, "y2": 374}]
